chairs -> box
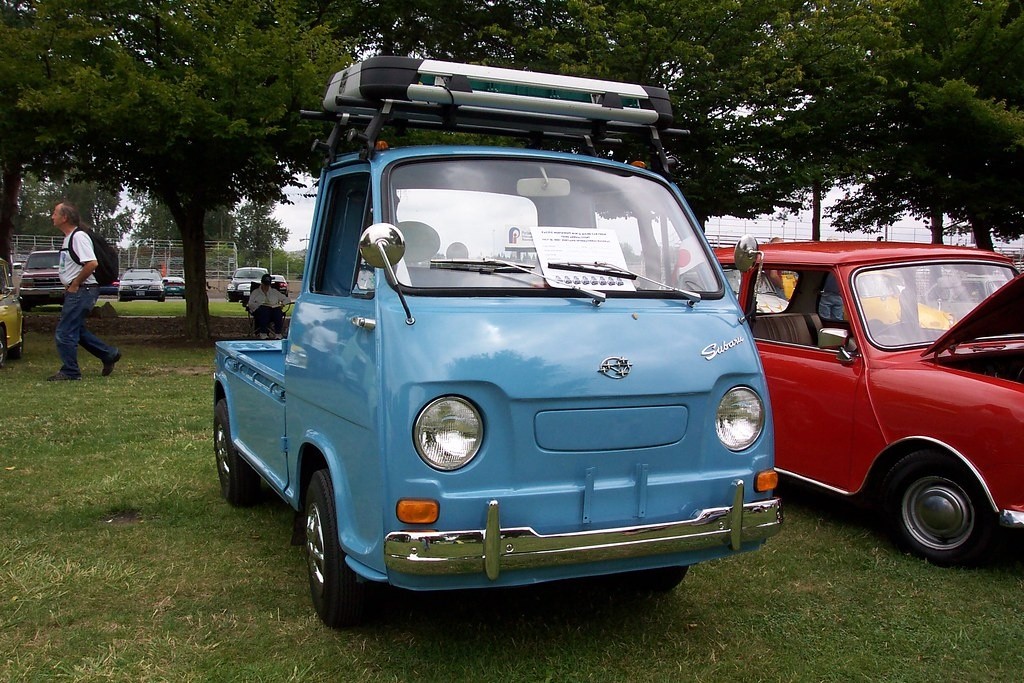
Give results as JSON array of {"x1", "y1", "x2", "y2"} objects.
[
  {"x1": 240, "y1": 282, "x2": 295, "y2": 340},
  {"x1": 394, "y1": 220, "x2": 441, "y2": 267}
]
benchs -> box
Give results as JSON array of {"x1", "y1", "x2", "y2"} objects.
[{"x1": 754, "y1": 312, "x2": 822, "y2": 346}]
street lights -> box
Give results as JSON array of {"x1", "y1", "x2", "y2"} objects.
[{"x1": 299, "y1": 239, "x2": 309, "y2": 250}]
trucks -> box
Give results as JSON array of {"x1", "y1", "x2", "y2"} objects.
[{"x1": 211, "y1": 56, "x2": 780, "y2": 629}]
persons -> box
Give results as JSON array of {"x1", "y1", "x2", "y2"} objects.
[
  {"x1": 818, "y1": 235, "x2": 844, "y2": 320},
  {"x1": 47, "y1": 201, "x2": 121, "y2": 380},
  {"x1": 248, "y1": 274, "x2": 291, "y2": 340},
  {"x1": 764, "y1": 238, "x2": 789, "y2": 301}
]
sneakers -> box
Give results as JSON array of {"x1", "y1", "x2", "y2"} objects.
[
  {"x1": 101, "y1": 348, "x2": 122, "y2": 376},
  {"x1": 46, "y1": 372, "x2": 82, "y2": 382},
  {"x1": 259, "y1": 333, "x2": 269, "y2": 340},
  {"x1": 274, "y1": 334, "x2": 283, "y2": 340}
]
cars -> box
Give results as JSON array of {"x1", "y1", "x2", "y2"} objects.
[
  {"x1": 12, "y1": 249, "x2": 66, "y2": 312},
  {"x1": 920, "y1": 273, "x2": 1010, "y2": 320},
  {"x1": 163, "y1": 277, "x2": 186, "y2": 299},
  {"x1": 226, "y1": 267, "x2": 270, "y2": 302},
  {"x1": 779, "y1": 270, "x2": 958, "y2": 333},
  {"x1": 663, "y1": 240, "x2": 1024, "y2": 569},
  {"x1": 0, "y1": 259, "x2": 24, "y2": 365},
  {"x1": 99, "y1": 279, "x2": 119, "y2": 295},
  {"x1": 118, "y1": 267, "x2": 169, "y2": 302},
  {"x1": 270, "y1": 275, "x2": 290, "y2": 297}
]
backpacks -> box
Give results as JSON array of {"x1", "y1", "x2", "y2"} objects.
[{"x1": 68, "y1": 227, "x2": 119, "y2": 287}]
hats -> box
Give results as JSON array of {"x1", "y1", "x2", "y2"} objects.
[{"x1": 262, "y1": 274, "x2": 272, "y2": 285}]
